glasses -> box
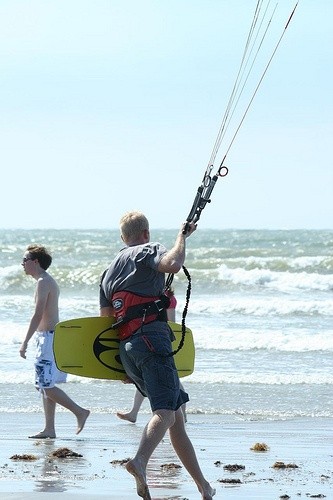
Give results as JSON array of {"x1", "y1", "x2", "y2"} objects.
[{"x1": 23, "y1": 258, "x2": 34, "y2": 263}]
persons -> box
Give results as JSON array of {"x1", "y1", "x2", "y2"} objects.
[
  {"x1": 19, "y1": 243, "x2": 90, "y2": 438},
  {"x1": 99, "y1": 211, "x2": 216, "y2": 500},
  {"x1": 117, "y1": 284, "x2": 187, "y2": 423}
]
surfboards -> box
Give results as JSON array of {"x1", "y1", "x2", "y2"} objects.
[{"x1": 52, "y1": 316, "x2": 195, "y2": 385}]
